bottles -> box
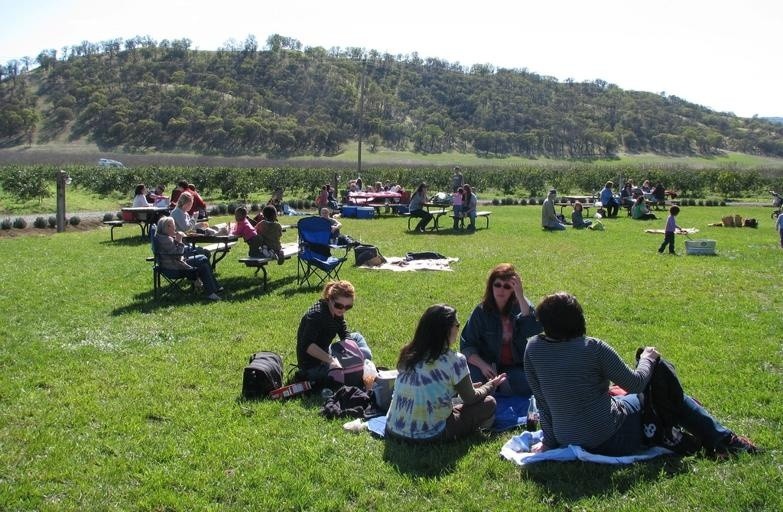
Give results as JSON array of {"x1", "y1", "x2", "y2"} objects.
[
  {"x1": 527, "y1": 395, "x2": 539, "y2": 432},
  {"x1": 488, "y1": 363, "x2": 498, "y2": 395}
]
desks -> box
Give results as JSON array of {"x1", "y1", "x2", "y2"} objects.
[
  {"x1": 123, "y1": 207, "x2": 171, "y2": 240},
  {"x1": 423, "y1": 202, "x2": 452, "y2": 229},
  {"x1": 183, "y1": 223, "x2": 291, "y2": 269},
  {"x1": 564, "y1": 195, "x2": 595, "y2": 217}
]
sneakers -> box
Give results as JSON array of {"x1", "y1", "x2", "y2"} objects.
[
  {"x1": 269, "y1": 250, "x2": 284, "y2": 265},
  {"x1": 210, "y1": 293, "x2": 223, "y2": 301},
  {"x1": 718, "y1": 434, "x2": 756, "y2": 462}
]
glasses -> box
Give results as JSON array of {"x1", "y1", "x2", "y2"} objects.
[
  {"x1": 493, "y1": 283, "x2": 512, "y2": 289},
  {"x1": 452, "y1": 321, "x2": 461, "y2": 328},
  {"x1": 333, "y1": 299, "x2": 353, "y2": 311}
]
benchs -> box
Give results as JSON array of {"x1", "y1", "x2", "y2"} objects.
[
  {"x1": 554, "y1": 203, "x2": 601, "y2": 218},
  {"x1": 238, "y1": 245, "x2": 299, "y2": 292},
  {"x1": 146, "y1": 241, "x2": 237, "y2": 271},
  {"x1": 449, "y1": 211, "x2": 493, "y2": 230},
  {"x1": 104, "y1": 220, "x2": 130, "y2": 242},
  {"x1": 406, "y1": 211, "x2": 450, "y2": 229}
]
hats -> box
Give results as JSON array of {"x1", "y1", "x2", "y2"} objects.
[{"x1": 419, "y1": 182, "x2": 430, "y2": 188}]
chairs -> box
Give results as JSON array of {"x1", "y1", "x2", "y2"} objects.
[
  {"x1": 297, "y1": 217, "x2": 347, "y2": 289},
  {"x1": 150, "y1": 223, "x2": 199, "y2": 302}
]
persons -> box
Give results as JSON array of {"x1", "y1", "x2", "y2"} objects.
[
  {"x1": 454, "y1": 187, "x2": 465, "y2": 231},
  {"x1": 461, "y1": 183, "x2": 476, "y2": 233},
  {"x1": 256, "y1": 206, "x2": 284, "y2": 266},
  {"x1": 524, "y1": 293, "x2": 764, "y2": 462},
  {"x1": 641, "y1": 180, "x2": 650, "y2": 192},
  {"x1": 460, "y1": 264, "x2": 538, "y2": 396},
  {"x1": 410, "y1": 184, "x2": 432, "y2": 233},
  {"x1": 629, "y1": 178, "x2": 637, "y2": 188},
  {"x1": 171, "y1": 192, "x2": 200, "y2": 233},
  {"x1": 571, "y1": 202, "x2": 592, "y2": 229},
  {"x1": 384, "y1": 303, "x2": 507, "y2": 445},
  {"x1": 601, "y1": 181, "x2": 619, "y2": 218},
  {"x1": 320, "y1": 208, "x2": 342, "y2": 241},
  {"x1": 296, "y1": 281, "x2": 371, "y2": 383},
  {"x1": 542, "y1": 189, "x2": 565, "y2": 231},
  {"x1": 631, "y1": 197, "x2": 654, "y2": 220},
  {"x1": 652, "y1": 181, "x2": 667, "y2": 210},
  {"x1": 156, "y1": 216, "x2": 224, "y2": 301},
  {"x1": 146, "y1": 184, "x2": 169, "y2": 206},
  {"x1": 453, "y1": 166, "x2": 464, "y2": 191},
  {"x1": 232, "y1": 207, "x2": 279, "y2": 261},
  {"x1": 622, "y1": 183, "x2": 634, "y2": 215},
  {"x1": 658, "y1": 205, "x2": 682, "y2": 255},
  {"x1": 775, "y1": 204, "x2": 783, "y2": 248},
  {"x1": 132, "y1": 184, "x2": 153, "y2": 208},
  {"x1": 171, "y1": 180, "x2": 206, "y2": 218},
  {"x1": 317, "y1": 178, "x2": 409, "y2": 217}
]
